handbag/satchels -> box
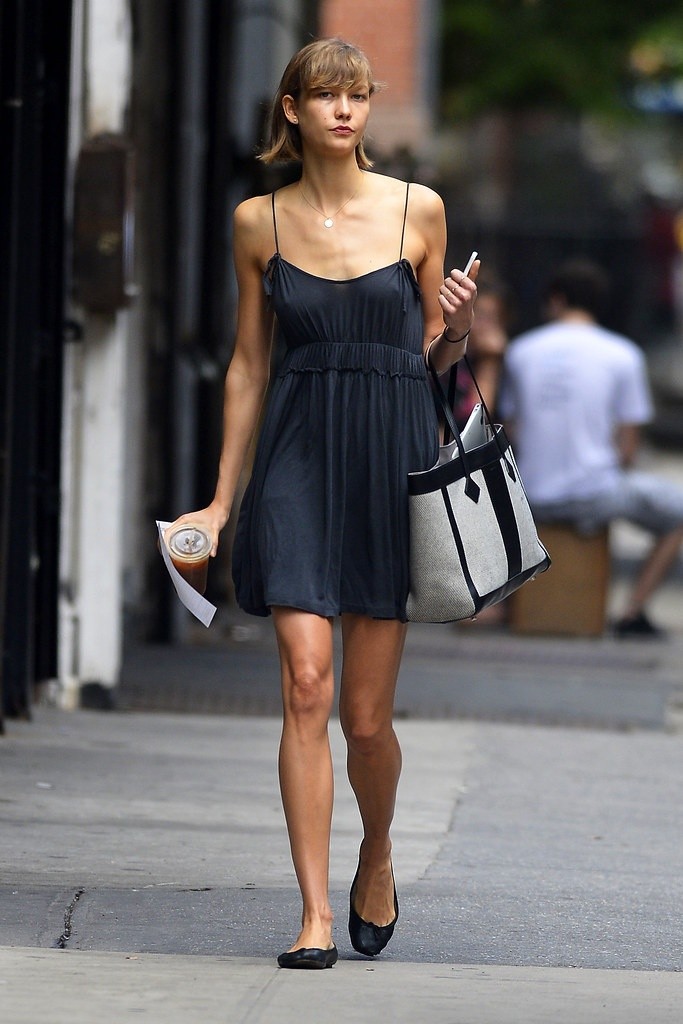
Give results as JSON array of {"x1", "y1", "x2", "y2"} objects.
[{"x1": 397, "y1": 344, "x2": 554, "y2": 623}]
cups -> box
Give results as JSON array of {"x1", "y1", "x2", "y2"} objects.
[{"x1": 167, "y1": 523, "x2": 210, "y2": 597}]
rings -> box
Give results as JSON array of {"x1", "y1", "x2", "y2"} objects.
[
  {"x1": 458, "y1": 275, "x2": 468, "y2": 286},
  {"x1": 451, "y1": 284, "x2": 461, "y2": 294}
]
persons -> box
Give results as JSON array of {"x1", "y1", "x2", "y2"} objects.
[
  {"x1": 507, "y1": 243, "x2": 683, "y2": 643},
  {"x1": 151, "y1": 32, "x2": 480, "y2": 972},
  {"x1": 437, "y1": 247, "x2": 505, "y2": 468}
]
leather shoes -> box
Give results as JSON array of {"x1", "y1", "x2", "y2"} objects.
[
  {"x1": 348, "y1": 837, "x2": 399, "y2": 958},
  {"x1": 277, "y1": 941, "x2": 338, "y2": 969}
]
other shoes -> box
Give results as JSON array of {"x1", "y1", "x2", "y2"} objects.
[{"x1": 614, "y1": 609, "x2": 670, "y2": 643}]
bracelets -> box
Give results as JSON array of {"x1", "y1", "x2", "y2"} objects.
[{"x1": 441, "y1": 322, "x2": 471, "y2": 344}]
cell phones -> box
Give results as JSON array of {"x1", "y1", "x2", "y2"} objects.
[{"x1": 465, "y1": 251, "x2": 479, "y2": 280}]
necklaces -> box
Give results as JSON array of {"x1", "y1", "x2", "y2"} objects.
[{"x1": 299, "y1": 169, "x2": 365, "y2": 229}]
